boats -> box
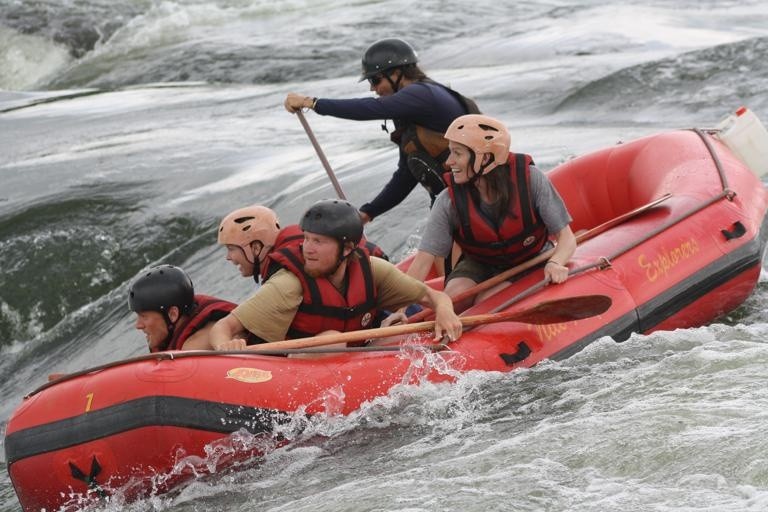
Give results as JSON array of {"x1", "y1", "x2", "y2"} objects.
[{"x1": 6, "y1": 127, "x2": 768, "y2": 512}]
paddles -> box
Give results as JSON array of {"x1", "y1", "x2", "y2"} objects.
[
  {"x1": 392, "y1": 190, "x2": 672, "y2": 326},
  {"x1": 244, "y1": 294, "x2": 613, "y2": 356}
]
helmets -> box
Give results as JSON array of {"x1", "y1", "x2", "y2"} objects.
[
  {"x1": 357, "y1": 38, "x2": 419, "y2": 92},
  {"x1": 443, "y1": 114, "x2": 511, "y2": 185},
  {"x1": 217, "y1": 205, "x2": 282, "y2": 284},
  {"x1": 127, "y1": 264, "x2": 195, "y2": 350},
  {"x1": 299, "y1": 198, "x2": 364, "y2": 274}
]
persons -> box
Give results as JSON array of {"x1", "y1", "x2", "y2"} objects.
[
  {"x1": 285, "y1": 39, "x2": 482, "y2": 225},
  {"x1": 210, "y1": 199, "x2": 462, "y2": 352},
  {"x1": 128, "y1": 264, "x2": 239, "y2": 353},
  {"x1": 379, "y1": 114, "x2": 576, "y2": 328},
  {"x1": 218, "y1": 206, "x2": 389, "y2": 285}
]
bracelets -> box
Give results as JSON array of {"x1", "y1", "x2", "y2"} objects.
[{"x1": 546, "y1": 261, "x2": 559, "y2": 266}]
